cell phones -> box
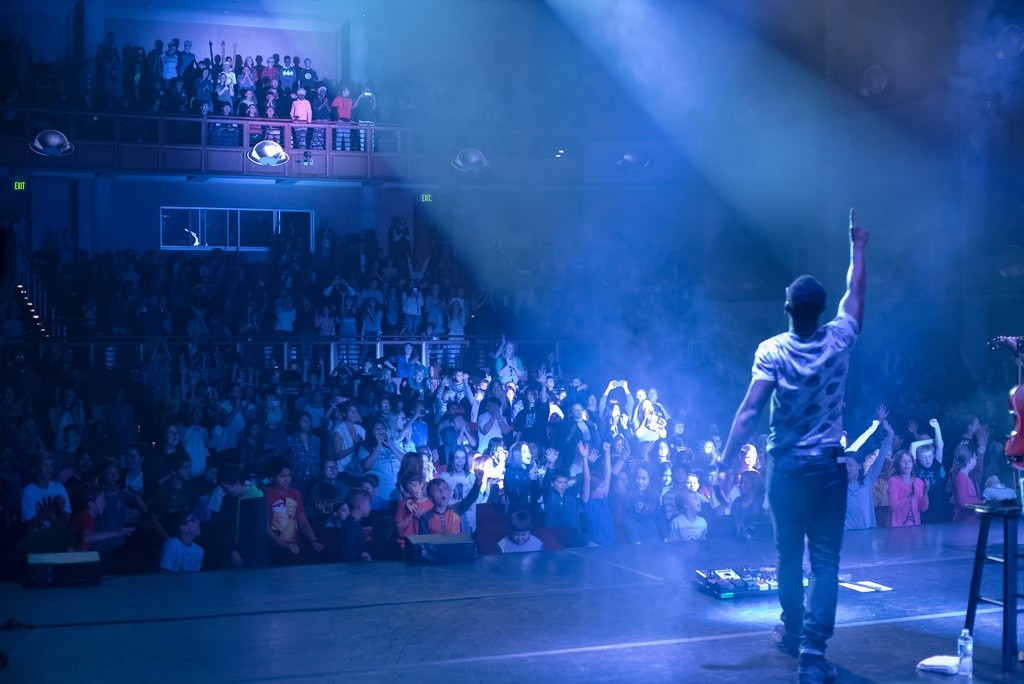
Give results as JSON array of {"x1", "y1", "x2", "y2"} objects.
[{"x1": 613, "y1": 381, "x2": 622, "y2": 387}]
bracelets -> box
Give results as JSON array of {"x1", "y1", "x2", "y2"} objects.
[{"x1": 716, "y1": 457, "x2": 732, "y2": 469}]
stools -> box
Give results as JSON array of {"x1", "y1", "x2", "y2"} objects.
[{"x1": 964, "y1": 500, "x2": 1023, "y2": 677}]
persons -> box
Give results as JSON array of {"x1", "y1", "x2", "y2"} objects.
[
  {"x1": 0, "y1": 216, "x2": 709, "y2": 575},
  {"x1": 696, "y1": 339, "x2": 1012, "y2": 539},
  {"x1": 0, "y1": 31, "x2": 433, "y2": 152},
  {"x1": 713, "y1": 208, "x2": 868, "y2": 684}
]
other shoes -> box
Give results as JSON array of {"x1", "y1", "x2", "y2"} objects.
[
  {"x1": 772, "y1": 624, "x2": 799, "y2": 655},
  {"x1": 797, "y1": 653, "x2": 838, "y2": 684}
]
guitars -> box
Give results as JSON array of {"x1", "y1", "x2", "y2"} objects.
[{"x1": 1004, "y1": 336, "x2": 1024, "y2": 471}]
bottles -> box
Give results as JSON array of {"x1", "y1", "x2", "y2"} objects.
[{"x1": 958, "y1": 630, "x2": 973, "y2": 676}]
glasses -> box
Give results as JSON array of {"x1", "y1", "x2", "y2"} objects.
[{"x1": 323, "y1": 466, "x2": 339, "y2": 471}]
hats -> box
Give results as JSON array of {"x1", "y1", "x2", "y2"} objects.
[{"x1": 297, "y1": 88, "x2": 306, "y2": 95}]
